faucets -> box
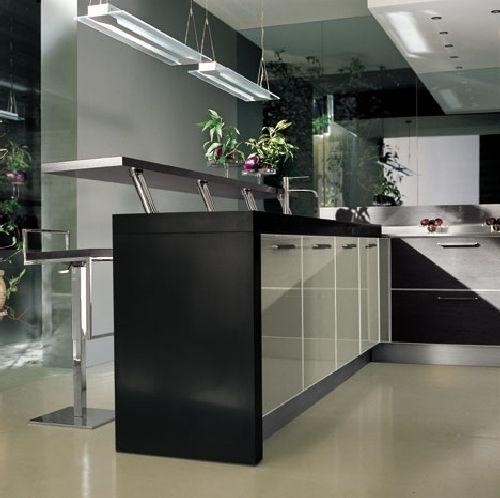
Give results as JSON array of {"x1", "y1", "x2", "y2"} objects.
[{"x1": 282, "y1": 174, "x2": 319, "y2": 214}]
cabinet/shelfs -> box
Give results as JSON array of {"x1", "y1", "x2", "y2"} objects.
[
  {"x1": 263, "y1": 236, "x2": 302, "y2": 420},
  {"x1": 381, "y1": 236, "x2": 500, "y2": 364},
  {"x1": 337, "y1": 235, "x2": 360, "y2": 373},
  {"x1": 361, "y1": 241, "x2": 379, "y2": 354},
  {"x1": 302, "y1": 237, "x2": 338, "y2": 391}
]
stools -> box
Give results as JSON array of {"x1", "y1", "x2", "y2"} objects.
[{"x1": 19, "y1": 227, "x2": 116, "y2": 428}]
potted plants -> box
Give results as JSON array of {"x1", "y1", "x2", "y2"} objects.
[{"x1": 200, "y1": 109, "x2": 300, "y2": 175}]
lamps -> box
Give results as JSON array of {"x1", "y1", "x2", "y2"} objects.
[{"x1": 74, "y1": 1, "x2": 279, "y2": 104}]
